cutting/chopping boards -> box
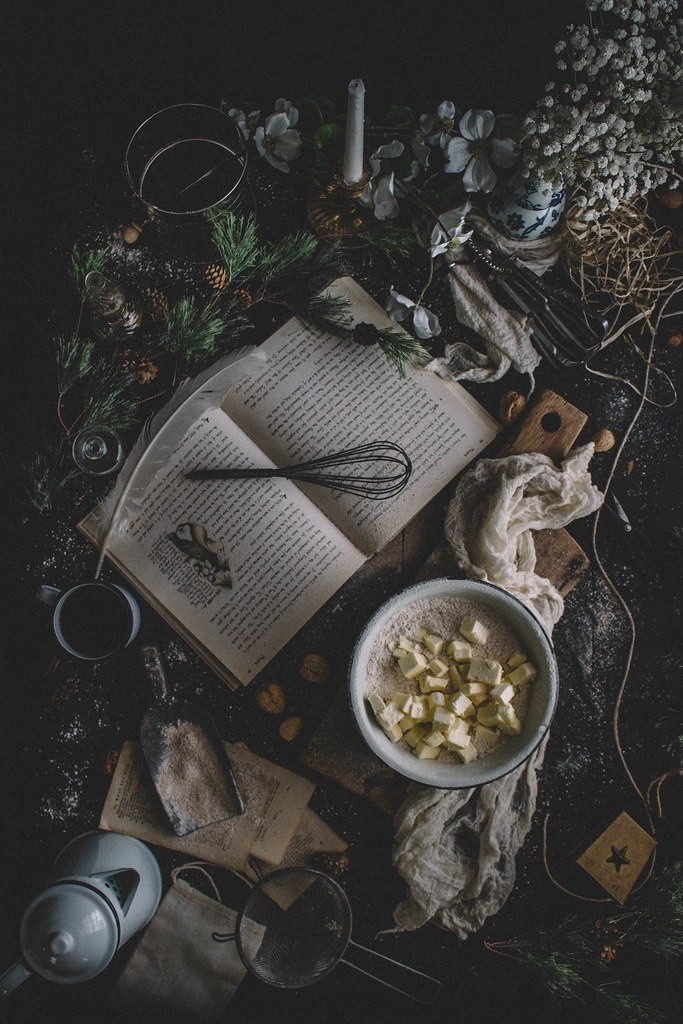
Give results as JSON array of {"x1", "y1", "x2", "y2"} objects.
[{"x1": 252, "y1": 388, "x2": 591, "y2": 817}]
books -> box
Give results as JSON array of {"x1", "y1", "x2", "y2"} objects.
[
  {"x1": 75, "y1": 275, "x2": 504, "y2": 692},
  {"x1": 95, "y1": 736, "x2": 352, "y2": 912}
]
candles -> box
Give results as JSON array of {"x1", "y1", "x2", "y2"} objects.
[{"x1": 343, "y1": 79, "x2": 366, "y2": 182}]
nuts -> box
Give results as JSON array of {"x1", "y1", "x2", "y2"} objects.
[
  {"x1": 254, "y1": 653, "x2": 331, "y2": 742},
  {"x1": 590, "y1": 429, "x2": 615, "y2": 452},
  {"x1": 499, "y1": 389, "x2": 526, "y2": 424}
]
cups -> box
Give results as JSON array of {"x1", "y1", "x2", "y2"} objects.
[{"x1": 35, "y1": 582, "x2": 141, "y2": 661}]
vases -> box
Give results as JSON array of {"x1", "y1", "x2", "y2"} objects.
[{"x1": 486, "y1": 153, "x2": 566, "y2": 240}]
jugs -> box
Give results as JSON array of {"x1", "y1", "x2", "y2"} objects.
[{"x1": 0, "y1": 831, "x2": 162, "y2": 1024}]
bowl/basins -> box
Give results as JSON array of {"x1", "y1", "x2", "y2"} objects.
[{"x1": 348, "y1": 578, "x2": 560, "y2": 789}]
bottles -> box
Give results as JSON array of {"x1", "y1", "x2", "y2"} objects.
[{"x1": 85, "y1": 271, "x2": 144, "y2": 336}]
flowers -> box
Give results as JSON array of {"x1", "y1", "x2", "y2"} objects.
[{"x1": 520, "y1": 0, "x2": 683, "y2": 221}]
